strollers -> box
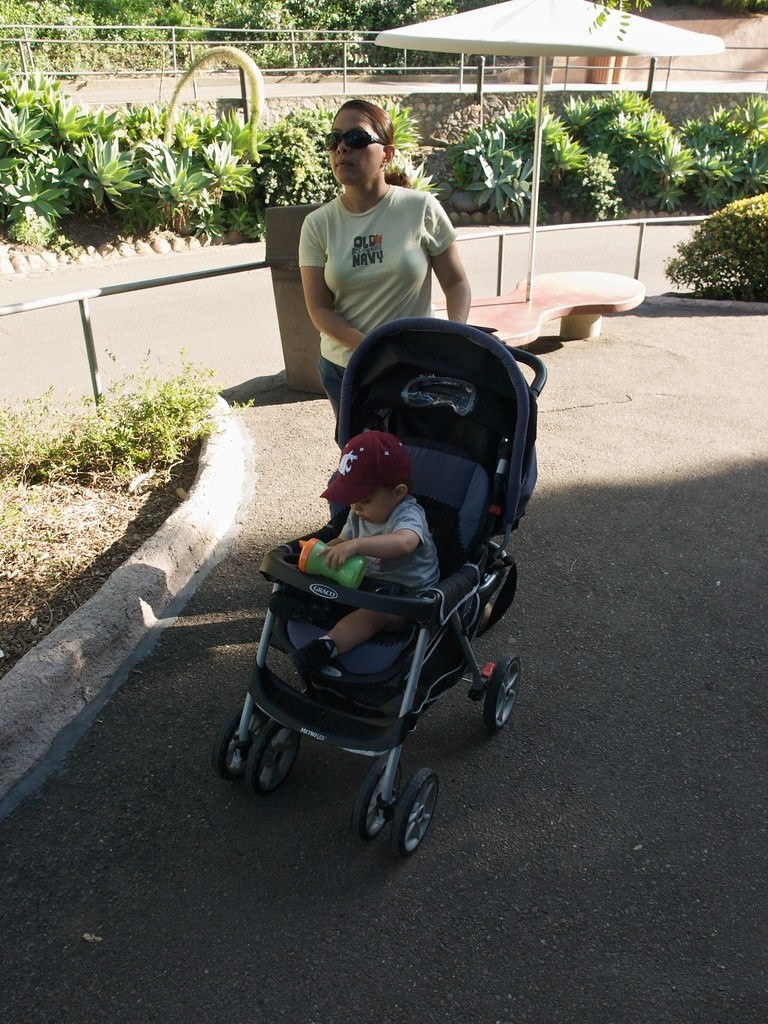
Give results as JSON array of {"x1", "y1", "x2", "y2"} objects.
[{"x1": 209, "y1": 317, "x2": 551, "y2": 858}]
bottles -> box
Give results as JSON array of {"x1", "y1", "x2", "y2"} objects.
[{"x1": 299, "y1": 538, "x2": 368, "y2": 589}]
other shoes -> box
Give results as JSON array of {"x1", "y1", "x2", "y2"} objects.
[
  {"x1": 269, "y1": 585, "x2": 331, "y2": 622},
  {"x1": 294, "y1": 638, "x2": 336, "y2": 681}
]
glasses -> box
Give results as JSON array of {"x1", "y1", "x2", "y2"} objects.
[{"x1": 325, "y1": 130, "x2": 386, "y2": 150}]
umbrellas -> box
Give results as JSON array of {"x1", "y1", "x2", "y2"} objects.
[{"x1": 376, "y1": 0, "x2": 725, "y2": 302}]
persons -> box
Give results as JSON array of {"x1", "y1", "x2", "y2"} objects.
[
  {"x1": 299, "y1": 99, "x2": 472, "y2": 445},
  {"x1": 267, "y1": 428, "x2": 442, "y2": 685}
]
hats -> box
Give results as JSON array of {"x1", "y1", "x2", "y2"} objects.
[{"x1": 320, "y1": 430, "x2": 412, "y2": 505}]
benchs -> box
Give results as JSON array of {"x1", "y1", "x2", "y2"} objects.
[{"x1": 430, "y1": 270, "x2": 646, "y2": 348}]
